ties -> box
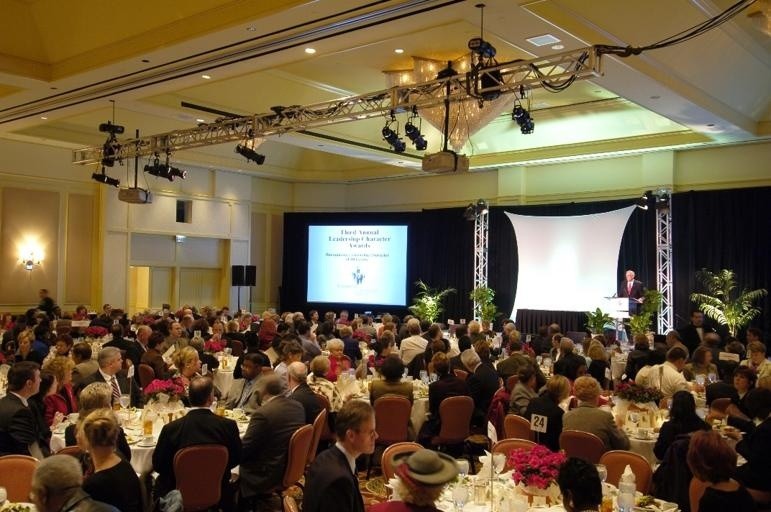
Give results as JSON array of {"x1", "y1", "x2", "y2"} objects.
[
  {"x1": 628, "y1": 283, "x2": 631, "y2": 295},
  {"x1": 111, "y1": 377, "x2": 121, "y2": 399}
]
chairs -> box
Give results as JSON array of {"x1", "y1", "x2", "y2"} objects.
[
  {"x1": 382, "y1": 443, "x2": 424, "y2": 500},
  {"x1": 314, "y1": 410, "x2": 326, "y2": 455},
  {"x1": 231, "y1": 341, "x2": 244, "y2": 356},
  {"x1": 690, "y1": 478, "x2": 713, "y2": 512},
  {"x1": 155, "y1": 444, "x2": 227, "y2": 508},
  {"x1": 505, "y1": 415, "x2": 535, "y2": 439},
  {"x1": 366, "y1": 395, "x2": 411, "y2": 481},
  {"x1": 56, "y1": 446, "x2": 86, "y2": 457},
  {"x1": 138, "y1": 364, "x2": 154, "y2": 392},
  {"x1": 599, "y1": 450, "x2": 652, "y2": 495},
  {"x1": 492, "y1": 439, "x2": 539, "y2": 474},
  {"x1": 2, "y1": 454, "x2": 39, "y2": 508},
  {"x1": 230, "y1": 424, "x2": 315, "y2": 508},
  {"x1": 491, "y1": 375, "x2": 516, "y2": 440},
  {"x1": 559, "y1": 430, "x2": 605, "y2": 465},
  {"x1": 430, "y1": 396, "x2": 473, "y2": 451},
  {"x1": 126, "y1": 358, "x2": 132, "y2": 368}
]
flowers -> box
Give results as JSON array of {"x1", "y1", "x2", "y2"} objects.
[
  {"x1": 85, "y1": 326, "x2": 107, "y2": 337},
  {"x1": 368, "y1": 355, "x2": 386, "y2": 372},
  {"x1": 525, "y1": 344, "x2": 536, "y2": 357},
  {"x1": 204, "y1": 339, "x2": 227, "y2": 352},
  {"x1": 615, "y1": 378, "x2": 665, "y2": 402},
  {"x1": 509, "y1": 446, "x2": 566, "y2": 490},
  {"x1": 351, "y1": 330, "x2": 370, "y2": 343},
  {"x1": 144, "y1": 380, "x2": 184, "y2": 402},
  {"x1": 506, "y1": 343, "x2": 511, "y2": 356}
]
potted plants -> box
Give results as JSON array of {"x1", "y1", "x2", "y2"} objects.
[
  {"x1": 584, "y1": 310, "x2": 616, "y2": 339},
  {"x1": 631, "y1": 310, "x2": 655, "y2": 350},
  {"x1": 413, "y1": 310, "x2": 435, "y2": 327},
  {"x1": 476, "y1": 310, "x2": 502, "y2": 332}
]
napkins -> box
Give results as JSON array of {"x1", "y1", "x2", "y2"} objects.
[{"x1": 478, "y1": 451, "x2": 506, "y2": 474}]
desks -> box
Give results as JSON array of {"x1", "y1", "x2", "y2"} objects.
[
  {"x1": 50, "y1": 406, "x2": 250, "y2": 477},
  {"x1": 360, "y1": 342, "x2": 402, "y2": 357},
  {"x1": 72, "y1": 338, "x2": 112, "y2": 360},
  {"x1": 560, "y1": 395, "x2": 748, "y2": 474},
  {"x1": 442, "y1": 332, "x2": 462, "y2": 358},
  {"x1": 536, "y1": 363, "x2": 556, "y2": 381},
  {"x1": 51, "y1": 321, "x2": 92, "y2": 330},
  {"x1": 372, "y1": 321, "x2": 386, "y2": 334},
  {"x1": 681, "y1": 380, "x2": 721, "y2": 407},
  {"x1": 491, "y1": 348, "x2": 515, "y2": 372},
  {"x1": 1, "y1": 364, "x2": 17, "y2": 396},
  {"x1": 435, "y1": 470, "x2": 678, "y2": 512},
  {"x1": 613, "y1": 352, "x2": 629, "y2": 378},
  {"x1": 329, "y1": 376, "x2": 433, "y2": 439},
  {"x1": 333, "y1": 379, "x2": 430, "y2": 434},
  {"x1": 214, "y1": 357, "x2": 243, "y2": 400}
]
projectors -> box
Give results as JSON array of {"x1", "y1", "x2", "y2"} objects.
[
  {"x1": 422, "y1": 152, "x2": 469, "y2": 175},
  {"x1": 118, "y1": 188, "x2": 152, "y2": 204}
]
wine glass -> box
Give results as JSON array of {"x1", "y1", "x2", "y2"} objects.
[
  {"x1": 73, "y1": 337, "x2": 103, "y2": 352},
  {"x1": 692, "y1": 372, "x2": 717, "y2": 397},
  {"x1": 601, "y1": 404, "x2": 670, "y2": 439},
  {"x1": 403, "y1": 366, "x2": 440, "y2": 384},
  {"x1": 114, "y1": 393, "x2": 251, "y2": 447},
  {"x1": 217, "y1": 346, "x2": 234, "y2": 371},
  {"x1": 337, "y1": 368, "x2": 372, "y2": 399},
  {"x1": 537, "y1": 355, "x2": 555, "y2": 375}
]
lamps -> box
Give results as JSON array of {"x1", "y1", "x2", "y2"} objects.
[
  {"x1": 379, "y1": 48, "x2": 539, "y2": 153},
  {"x1": 462, "y1": 198, "x2": 488, "y2": 221},
  {"x1": 70, "y1": 4, "x2": 605, "y2": 188},
  {"x1": 635, "y1": 188, "x2": 671, "y2": 211},
  {"x1": 20, "y1": 247, "x2": 41, "y2": 272}
]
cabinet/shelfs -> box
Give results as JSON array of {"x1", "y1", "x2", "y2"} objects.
[{"x1": 602, "y1": 310, "x2": 636, "y2": 347}]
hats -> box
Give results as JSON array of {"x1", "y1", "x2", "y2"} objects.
[{"x1": 391, "y1": 449, "x2": 460, "y2": 487}]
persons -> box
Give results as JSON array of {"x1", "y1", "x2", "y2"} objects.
[{"x1": 618, "y1": 270, "x2": 649, "y2": 304}]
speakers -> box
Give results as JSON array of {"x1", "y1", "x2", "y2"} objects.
[
  {"x1": 245, "y1": 266, "x2": 256, "y2": 286},
  {"x1": 232, "y1": 265, "x2": 244, "y2": 286}
]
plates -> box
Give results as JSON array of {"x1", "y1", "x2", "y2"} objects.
[
  {"x1": 636, "y1": 494, "x2": 679, "y2": 512},
  {"x1": 9, "y1": 501, "x2": 38, "y2": 512}
]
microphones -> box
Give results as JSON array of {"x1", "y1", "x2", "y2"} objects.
[{"x1": 612, "y1": 284, "x2": 627, "y2": 297}]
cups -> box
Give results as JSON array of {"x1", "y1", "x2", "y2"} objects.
[
  {"x1": 439, "y1": 458, "x2": 636, "y2": 512},
  {"x1": 54, "y1": 412, "x2": 80, "y2": 434},
  {"x1": 194, "y1": 330, "x2": 202, "y2": 337},
  {"x1": 1, "y1": 486, "x2": 8, "y2": 507}
]
